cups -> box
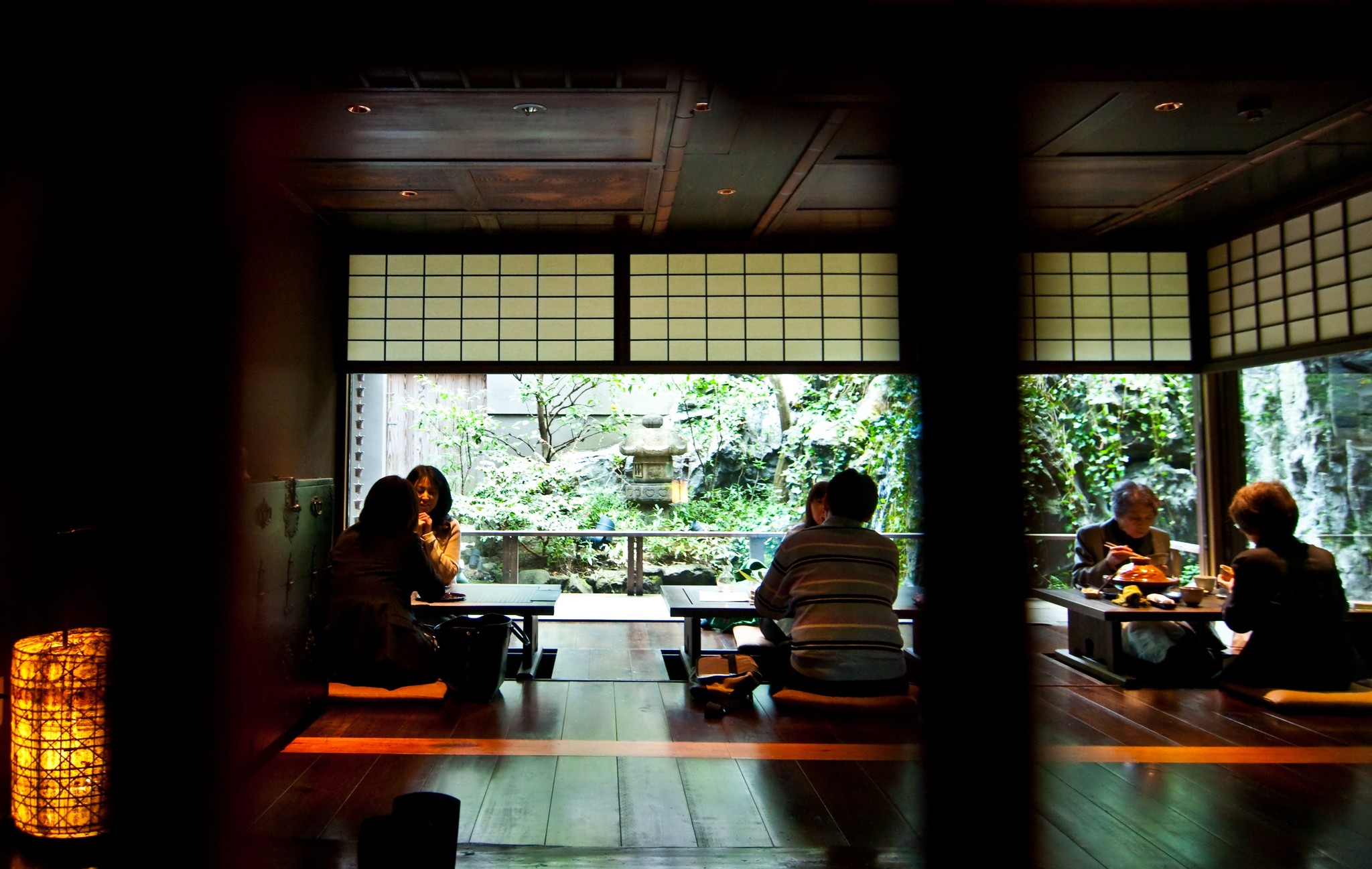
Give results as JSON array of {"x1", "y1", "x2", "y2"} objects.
[
  {"x1": 1179, "y1": 587, "x2": 1204, "y2": 606},
  {"x1": 1193, "y1": 575, "x2": 1216, "y2": 593}
]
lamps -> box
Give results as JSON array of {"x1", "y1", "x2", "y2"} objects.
[
  {"x1": 671, "y1": 477, "x2": 688, "y2": 502},
  {"x1": 11, "y1": 623, "x2": 111, "y2": 837}
]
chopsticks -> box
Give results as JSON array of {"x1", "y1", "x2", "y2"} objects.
[{"x1": 1104, "y1": 542, "x2": 1143, "y2": 557}]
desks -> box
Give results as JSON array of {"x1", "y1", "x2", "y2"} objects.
[
  {"x1": 411, "y1": 584, "x2": 561, "y2": 680},
  {"x1": 1028, "y1": 587, "x2": 1372, "y2": 690},
  {"x1": 660, "y1": 585, "x2": 917, "y2": 677}
]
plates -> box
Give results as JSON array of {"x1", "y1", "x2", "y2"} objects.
[
  {"x1": 415, "y1": 593, "x2": 466, "y2": 601},
  {"x1": 1216, "y1": 594, "x2": 1227, "y2": 597},
  {"x1": 1101, "y1": 588, "x2": 1181, "y2": 601}
]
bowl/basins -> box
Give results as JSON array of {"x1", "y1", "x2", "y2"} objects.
[
  {"x1": 1102, "y1": 556, "x2": 1179, "y2": 595},
  {"x1": 1219, "y1": 564, "x2": 1235, "y2": 581},
  {"x1": 444, "y1": 586, "x2": 455, "y2": 596},
  {"x1": 1146, "y1": 553, "x2": 1169, "y2": 566}
]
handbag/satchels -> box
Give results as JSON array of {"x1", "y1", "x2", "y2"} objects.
[{"x1": 421, "y1": 614, "x2": 531, "y2": 705}]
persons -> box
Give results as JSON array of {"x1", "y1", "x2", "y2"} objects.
[
  {"x1": 1068, "y1": 482, "x2": 1353, "y2": 691},
  {"x1": 753, "y1": 468, "x2": 908, "y2": 697},
  {"x1": 306, "y1": 464, "x2": 462, "y2": 691}
]
